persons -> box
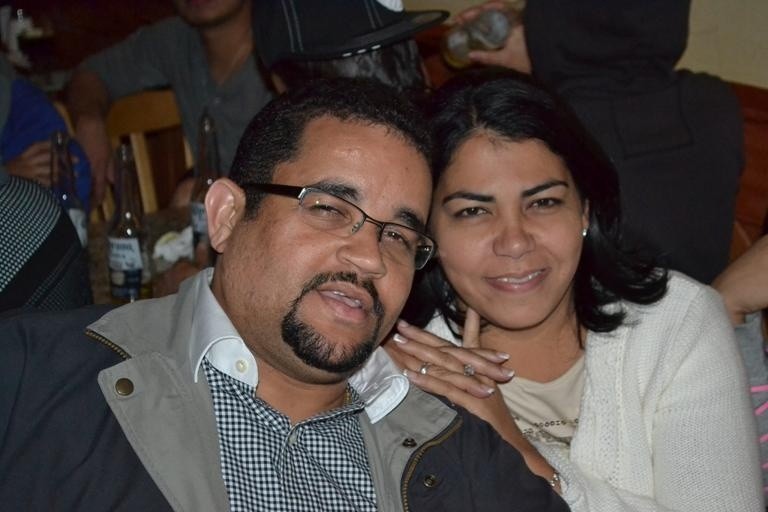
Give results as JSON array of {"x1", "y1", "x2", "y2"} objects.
[
  {"x1": 0, "y1": 164, "x2": 97, "y2": 313},
  {"x1": 371, "y1": 63, "x2": 763, "y2": 512},
  {"x1": 248, "y1": 0, "x2": 450, "y2": 112},
  {"x1": 65, "y1": 0, "x2": 282, "y2": 203},
  {"x1": 446, "y1": 0, "x2": 745, "y2": 286},
  {"x1": 0, "y1": 72, "x2": 571, "y2": 512},
  {"x1": 711, "y1": 231, "x2": 768, "y2": 507},
  {"x1": 0, "y1": 48, "x2": 94, "y2": 250}
]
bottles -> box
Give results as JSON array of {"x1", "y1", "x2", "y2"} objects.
[
  {"x1": 188, "y1": 108, "x2": 228, "y2": 270},
  {"x1": 104, "y1": 141, "x2": 151, "y2": 306},
  {"x1": 435, "y1": 6, "x2": 512, "y2": 79},
  {"x1": 43, "y1": 130, "x2": 93, "y2": 259}
]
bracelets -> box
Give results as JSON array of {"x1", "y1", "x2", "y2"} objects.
[{"x1": 547, "y1": 471, "x2": 561, "y2": 488}]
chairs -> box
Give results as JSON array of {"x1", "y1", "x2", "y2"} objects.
[{"x1": 82, "y1": 86, "x2": 198, "y2": 225}]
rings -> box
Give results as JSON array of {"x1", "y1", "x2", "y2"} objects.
[
  {"x1": 462, "y1": 363, "x2": 474, "y2": 376},
  {"x1": 417, "y1": 361, "x2": 431, "y2": 374}
]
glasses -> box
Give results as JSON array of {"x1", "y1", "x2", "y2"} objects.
[{"x1": 242, "y1": 181, "x2": 438, "y2": 269}]
